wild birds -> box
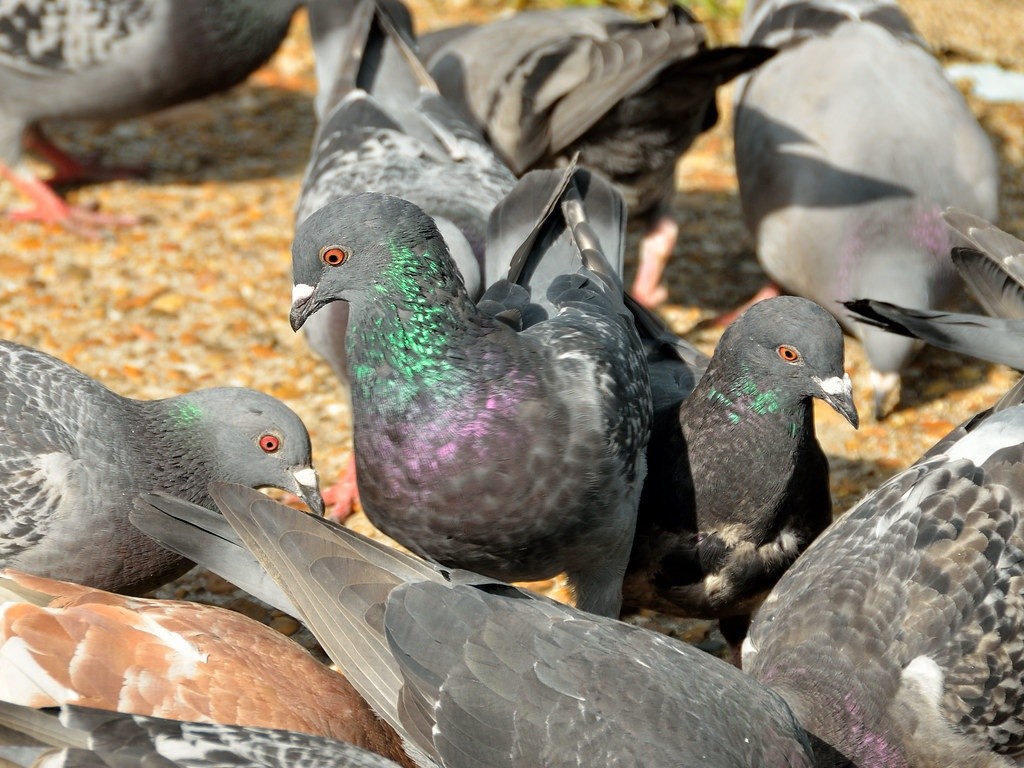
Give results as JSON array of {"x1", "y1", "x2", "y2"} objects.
[
  {"x1": 0, "y1": 1, "x2": 1024, "y2": 524},
  {"x1": 127, "y1": 149, "x2": 1024, "y2": 768},
  {"x1": 0, "y1": 341, "x2": 419, "y2": 768}
]
